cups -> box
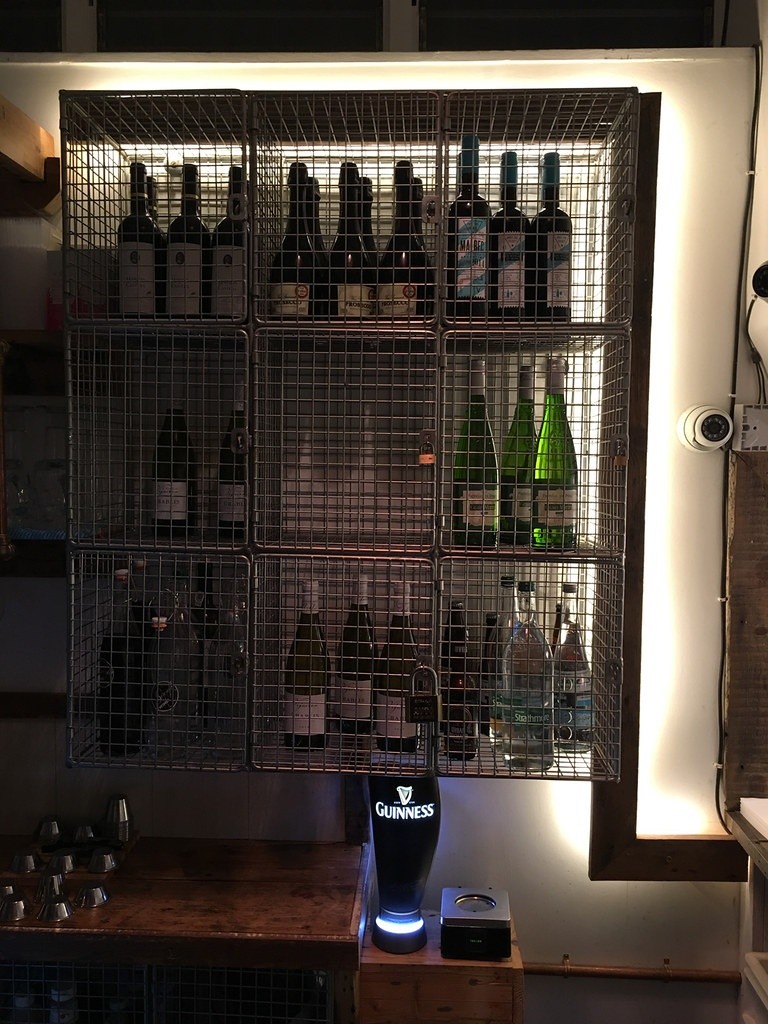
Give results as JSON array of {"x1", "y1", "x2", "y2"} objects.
[{"x1": 31, "y1": 427, "x2": 67, "y2": 530}]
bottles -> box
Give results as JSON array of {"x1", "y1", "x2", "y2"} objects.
[
  {"x1": 336, "y1": 574, "x2": 379, "y2": 734},
  {"x1": 218, "y1": 380, "x2": 247, "y2": 540},
  {"x1": 499, "y1": 367, "x2": 538, "y2": 545},
  {"x1": 452, "y1": 359, "x2": 500, "y2": 549},
  {"x1": 440, "y1": 601, "x2": 471, "y2": 731},
  {"x1": 270, "y1": 161, "x2": 433, "y2": 323},
  {"x1": 482, "y1": 576, "x2": 596, "y2": 772},
  {"x1": 492, "y1": 152, "x2": 530, "y2": 326},
  {"x1": 5, "y1": 430, "x2": 29, "y2": 528},
  {"x1": 211, "y1": 167, "x2": 252, "y2": 324},
  {"x1": 530, "y1": 153, "x2": 571, "y2": 326},
  {"x1": 377, "y1": 582, "x2": 418, "y2": 752},
  {"x1": 531, "y1": 359, "x2": 577, "y2": 551},
  {"x1": 449, "y1": 136, "x2": 492, "y2": 325},
  {"x1": 10, "y1": 978, "x2": 131, "y2": 1024},
  {"x1": 284, "y1": 581, "x2": 330, "y2": 751},
  {"x1": 167, "y1": 165, "x2": 211, "y2": 319},
  {"x1": 153, "y1": 394, "x2": 197, "y2": 537},
  {"x1": 118, "y1": 163, "x2": 166, "y2": 319},
  {"x1": 100, "y1": 561, "x2": 249, "y2": 754},
  {"x1": 446, "y1": 646, "x2": 479, "y2": 761}
]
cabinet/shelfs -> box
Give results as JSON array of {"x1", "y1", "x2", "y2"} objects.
[{"x1": 0, "y1": 828, "x2": 529, "y2": 1024}]
